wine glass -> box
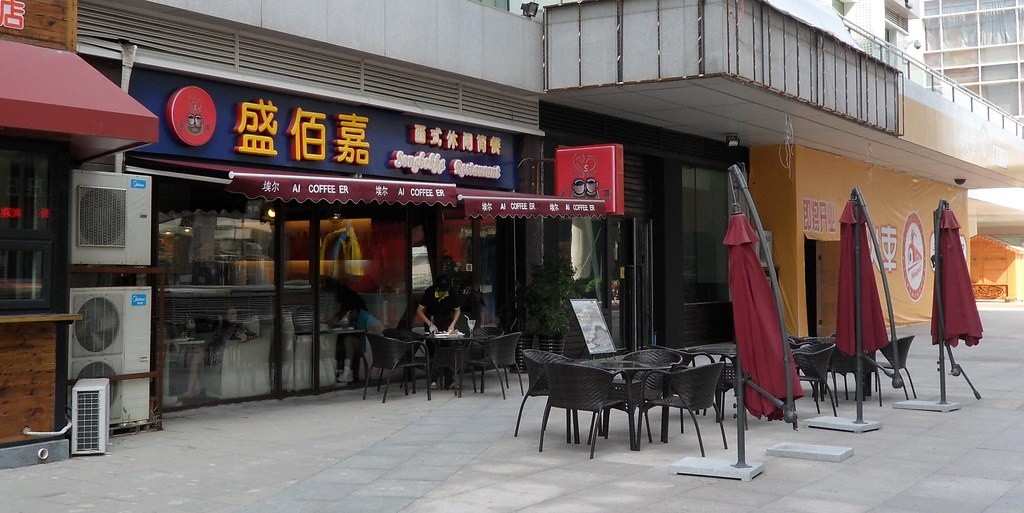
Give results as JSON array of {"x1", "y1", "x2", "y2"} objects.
[
  {"x1": 185, "y1": 320, "x2": 195, "y2": 340},
  {"x1": 467, "y1": 320, "x2": 476, "y2": 338}
]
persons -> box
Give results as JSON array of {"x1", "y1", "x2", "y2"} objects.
[
  {"x1": 178, "y1": 306, "x2": 257, "y2": 398},
  {"x1": 325, "y1": 278, "x2": 369, "y2": 382},
  {"x1": 416, "y1": 275, "x2": 462, "y2": 390}
]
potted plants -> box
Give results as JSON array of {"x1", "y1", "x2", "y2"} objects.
[
  {"x1": 509, "y1": 283, "x2": 533, "y2": 372},
  {"x1": 530, "y1": 251, "x2": 576, "y2": 355}
]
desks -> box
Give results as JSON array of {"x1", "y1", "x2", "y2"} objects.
[
  {"x1": 401, "y1": 332, "x2": 486, "y2": 398},
  {"x1": 573, "y1": 358, "x2": 672, "y2": 450},
  {"x1": 688, "y1": 344, "x2": 738, "y2": 422},
  {"x1": 804, "y1": 337, "x2": 872, "y2": 401},
  {"x1": 291, "y1": 328, "x2": 366, "y2": 386},
  {"x1": 164, "y1": 336, "x2": 205, "y2": 406}
]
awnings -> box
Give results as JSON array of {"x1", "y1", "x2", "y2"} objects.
[
  {"x1": 0, "y1": 40, "x2": 159, "y2": 163},
  {"x1": 413, "y1": 188, "x2": 606, "y2": 220},
  {"x1": 127, "y1": 154, "x2": 456, "y2": 215}
]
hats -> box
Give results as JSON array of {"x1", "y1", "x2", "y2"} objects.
[{"x1": 436, "y1": 273, "x2": 449, "y2": 292}]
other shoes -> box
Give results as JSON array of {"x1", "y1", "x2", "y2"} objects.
[
  {"x1": 338, "y1": 368, "x2": 354, "y2": 382},
  {"x1": 449, "y1": 382, "x2": 459, "y2": 389},
  {"x1": 430, "y1": 381, "x2": 437, "y2": 390}
]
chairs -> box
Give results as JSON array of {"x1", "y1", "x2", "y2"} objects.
[
  {"x1": 604, "y1": 350, "x2": 684, "y2": 442},
  {"x1": 459, "y1": 327, "x2": 510, "y2": 388},
  {"x1": 779, "y1": 342, "x2": 837, "y2": 421},
  {"x1": 515, "y1": 350, "x2": 575, "y2": 443},
  {"x1": 720, "y1": 354, "x2": 772, "y2": 422},
  {"x1": 638, "y1": 361, "x2": 728, "y2": 458},
  {"x1": 649, "y1": 344, "x2": 715, "y2": 415},
  {"x1": 831, "y1": 333, "x2": 878, "y2": 393},
  {"x1": 787, "y1": 334, "x2": 825, "y2": 401},
  {"x1": 206, "y1": 315, "x2": 274, "y2": 399},
  {"x1": 456, "y1": 332, "x2": 525, "y2": 400},
  {"x1": 854, "y1": 334, "x2": 917, "y2": 407},
  {"x1": 362, "y1": 333, "x2": 408, "y2": 396},
  {"x1": 380, "y1": 329, "x2": 432, "y2": 401},
  {"x1": 270, "y1": 312, "x2": 335, "y2": 391},
  {"x1": 539, "y1": 363, "x2": 637, "y2": 459}
]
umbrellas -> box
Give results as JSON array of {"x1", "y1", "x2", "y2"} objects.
[
  {"x1": 930, "y1": 199, "x2": 983, "y2": 402},
  {"x1": 836, "y1": 188, "x2": 887, "y2": 421},
  {"x1": 723, "y1": 165, "x2": 804, "y2": 463}
]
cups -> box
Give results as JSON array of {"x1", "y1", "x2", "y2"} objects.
[
  {"x1": 342, "y1": 318, "x2": 348, "y2": 331},
  {"x1": 586, "y1": 179, "x2": 599, "y2": 192},
  {"x1": 572, "y1": 180, "x2": 584, "y2": 194}
]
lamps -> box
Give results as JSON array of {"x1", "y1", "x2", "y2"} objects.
[
  {"x1": 726, "y1": 132, "x2": 739, "y2": 147},
  {"x1": 521, "y1": 2, "x2": 540, "y2": 18}
]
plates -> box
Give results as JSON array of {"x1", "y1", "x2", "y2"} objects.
[
  {"x1": 461, "y1": 333, "x2": 465, "y2": 337},
  {"x1": 434, "y1": 333, "x2": 448, "y2": 337},
  {"x1": 586, "y1": 190, "x2": 597, "y2": 196},
  {"x1": 573, "y1": 192, "x2": 585, "y2": 197}
]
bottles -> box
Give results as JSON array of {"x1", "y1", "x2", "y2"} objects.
[{"x1": 429, "y1": 316, "x2": 435, "y2": 339}]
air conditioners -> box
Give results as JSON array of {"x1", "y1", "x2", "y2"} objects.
[
  {"x1": 72, "y1": 379, "x2": 110, "y2": 454},
  {"x1": 69, "y1": 284, "x2": 149, "y2": 424},
  {"x1": 70, "y1": 169, "x2": 151, "y2": 265}
]
